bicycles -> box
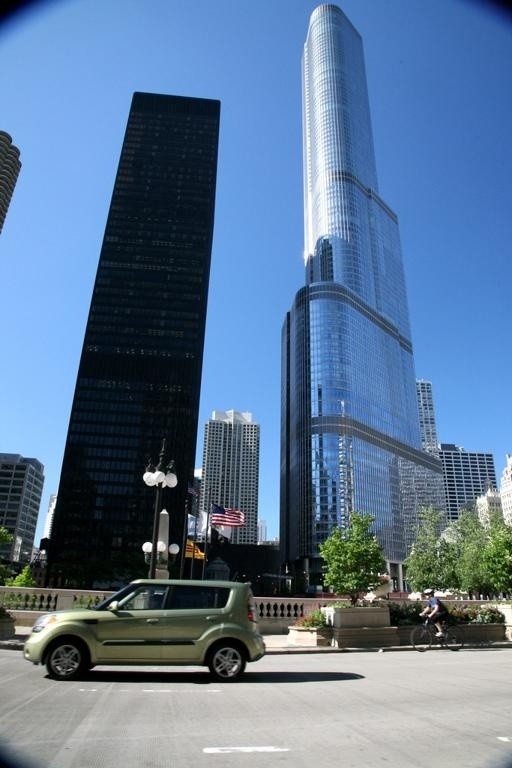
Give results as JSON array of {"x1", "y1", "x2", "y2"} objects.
[{"x1": 411, "y1": 613, "x2": 466, "y2": 652}]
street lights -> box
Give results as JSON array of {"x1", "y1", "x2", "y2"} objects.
[
  {"x1": 141, "y1": 434, "x2": 185, "y2": 579},
  {"x1": 142, "y1": 541, "x2": 181, "y2": 571}
]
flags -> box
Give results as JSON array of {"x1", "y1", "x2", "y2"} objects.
[{"x1": 185, "y1": 503, "x2": 246, "y2": 562}]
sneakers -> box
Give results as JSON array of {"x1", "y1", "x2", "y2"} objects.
[{"x1": 435, "y1": 631, "x2": 445, "y2": 638}]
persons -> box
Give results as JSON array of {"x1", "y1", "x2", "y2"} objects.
[{"x1": 419, "y1": 588, "x2": 450, "y2": 638}]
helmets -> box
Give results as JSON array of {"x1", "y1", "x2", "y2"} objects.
[{"x1": 423, "y1": 588, "x2": 434, "y2": 595}]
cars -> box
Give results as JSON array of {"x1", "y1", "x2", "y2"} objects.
[{"x1": 22, "y1": 579, "x2": 266, "y2": 683}]
